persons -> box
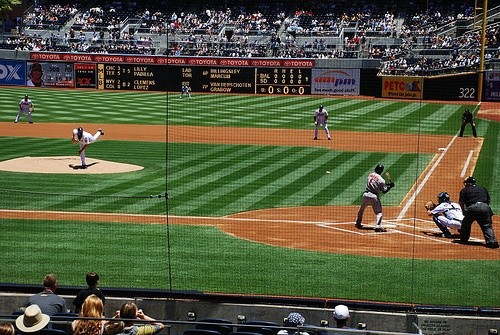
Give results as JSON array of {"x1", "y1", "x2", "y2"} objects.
[
  {"x1": 27, "y1": 63, "x2": 46, "y2": 87},
  {"x1": 354, "y1": 163, "x2": 394, "y2": 232},
  {"x1": 314, "y1": 105, "x2": 331, "y2": 140},
  {"x1": 179, "y1": 84, "x2": 192, "y2": 99},
  {"x1": 321, "y1": 305, "x2": 362, "y2": 335},
  {"x1": 425, "y1": 191, "x2": 466, "y2": 238},
  {"x1": 72, "y1": 126, "x2": 104, "y2": 168},
  {"x1": 73, "y1": 272, "x2": 106, "y2": 317},
  {"x1": 455, "y1": 177, "x2": 499, "y2": 249},
  {"x1": 15, "y1": 94, "x2": 35, "y2": 124},
  {"x1": 23, "y1": 275, "x2": 70, "y2": 334},
  {"x1": 105, "y1": 302, "x2": 165, "y2": 335},
  {"x1": 0, "y1": 322, "x2": 15, "y2": 335},
  {"x1": 0, "y1": 2, "x2": 381, "y2": 62},
  {"x1": 459, "y1": 109, "x2": 478, "y2": 137},
  {"x1": 277, "y1": 313, "x2": 310, "y2": 335},
  {"x1": 381, "y1": 0, "x2": 500, "y2": 76},
  {"x1": 70, "y1": 295, "x2": 106, "y2": 335}
]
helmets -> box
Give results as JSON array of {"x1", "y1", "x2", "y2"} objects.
[
  {"x1": 437, "y1": 191, "x2": 450, "y2": 201},
  {"x1": 463, "y1": 176, "x2": 476, "y2": 187},
  {"x1": 376, "y1": 164, "x2": 385, "y2": 171},
  {"x1": 25, "y1": 94, "x2": 29, "y2": 97},
  {"x1": 465, "y1": 108, "x2": 469, "y2": 113},
  {"x1": 320, "y1": 105, "x2": 323, "y2": 108},
  {"x1": 77, "y1": 127, "x2": 83, "y2": 131}
]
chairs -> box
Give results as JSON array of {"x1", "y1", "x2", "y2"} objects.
[
  {"x1": 195, "y1": 318, "x2": 233, "y2": 334},
  {"x1": 51, "y1": 312, "x2": 80, "y2": 321},
  {"x1": 183, "y1": 329, "x2": 221, "y2": 335},
  {"x1": 228, "y1": 332, "x2": 264, "y2": 335},
  {"x1": 39, "y1": 329, "x2": 66, "y2": 335},
  {"x1": 237, "y1": 321, "x2": 281, "y2": 334},
  {"x1": 11, "y1": 310, "x2": 25, "y2": 319}
]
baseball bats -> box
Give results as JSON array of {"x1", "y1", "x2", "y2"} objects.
[{"x1": 385, "y1": 172, "x2": 391, "y2": 183}]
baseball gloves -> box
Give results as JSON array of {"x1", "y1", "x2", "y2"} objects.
[{"x1": 425, "y1": 200, "x2": 437, "y2": 210}]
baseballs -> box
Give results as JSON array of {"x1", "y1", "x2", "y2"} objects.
[{"x1": 326, "y1": 170, "x2": 331, "y2": 174}]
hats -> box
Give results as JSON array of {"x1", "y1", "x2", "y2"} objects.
[
  {"x1": 284, "y1": 313, "x2": 305, "y2": 325},
  {"x1": 32, "y1": 64, "x2": 43, "y2": 71},
  {"x1": 333, "y1": 304, "x2": 351, "y2": 319}
]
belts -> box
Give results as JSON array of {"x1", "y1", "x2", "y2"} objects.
[
  {"x1": 450, "y1": 218, "x2": 460, "y2": 221},
  {"x1": 468, "y1": 200, "x2": 486, "y2": 206}
]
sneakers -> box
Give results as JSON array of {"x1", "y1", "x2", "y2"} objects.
[
  {"x1": 459, "y1": 239, "x2": 469, "y2": 244},
  {"x1": 314, "y1": 137, "x2": 318, "y2": 140},
  {"x1": 29, "y1": 122, "x2": 33, "y2": 124},
  {"x1": 375, "y1": 227, "x2": 386, "y2": 232},
  {"x1": 489, "y1": 242, "x2": 499, "y2": 248},
  {"x1": 474, "y1": 135, "x2": 477, "y2": 137},
  {"x1": 98, "y1": 128, "x2": 105, "y2": 135},
  {"x1": 355, "y1": 221, "x2": 364, "y2": 229},
  {"x1": 81, "y1": 164, "x2": 86, "y2": 168},
  {"x1": 439, "y1": 232, "x2": 452, "y2": 238},
  {"x1": 458, "y1": 134, "x2": 463, "y2": 137},
  {"x1": 328, "y1": 137, "x2": 331, "y2": 140},
  {"x1": 15, "y1": 120, "x2": 17, "y2": 122}
]
